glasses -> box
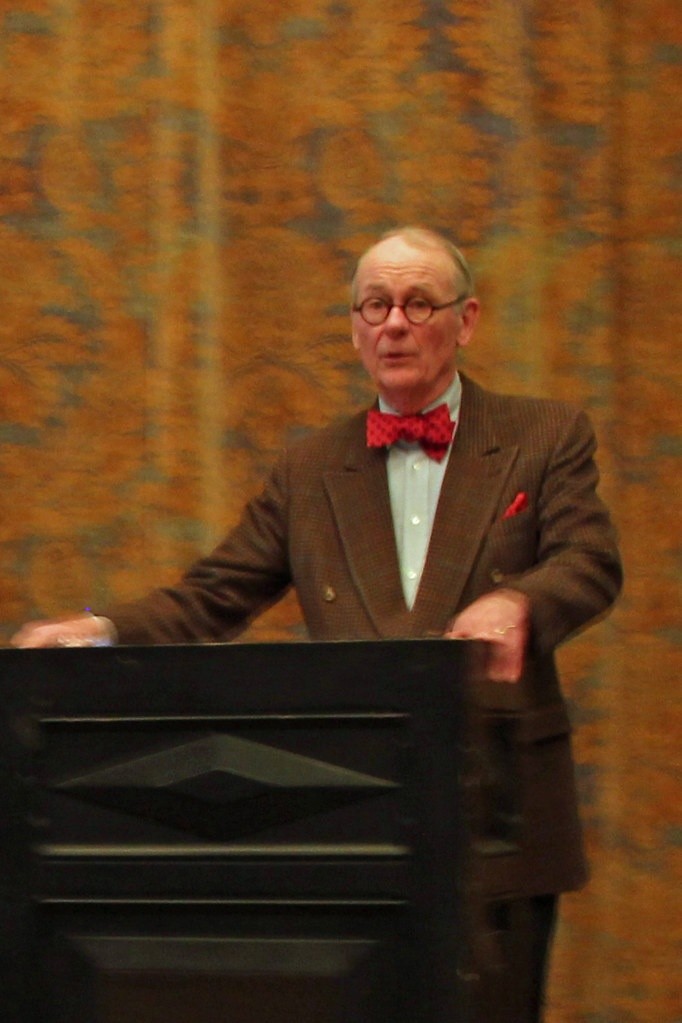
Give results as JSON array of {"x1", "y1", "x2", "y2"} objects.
[{"x1": 353, "y1": 292, "x2": 466, "y2": 326}]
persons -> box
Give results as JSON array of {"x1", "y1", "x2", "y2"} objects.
[{"x1": 11, "y1": 227, "x2": 625, "y2": 1023}]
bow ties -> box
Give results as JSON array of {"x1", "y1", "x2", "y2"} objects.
[{"x1": 366, "y1": 404, "x2": 456, "y2": 464}]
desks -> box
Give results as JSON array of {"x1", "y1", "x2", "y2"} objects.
[{"x1": 0, "y1": 639, "x2": 489, "y2": 1022}]
